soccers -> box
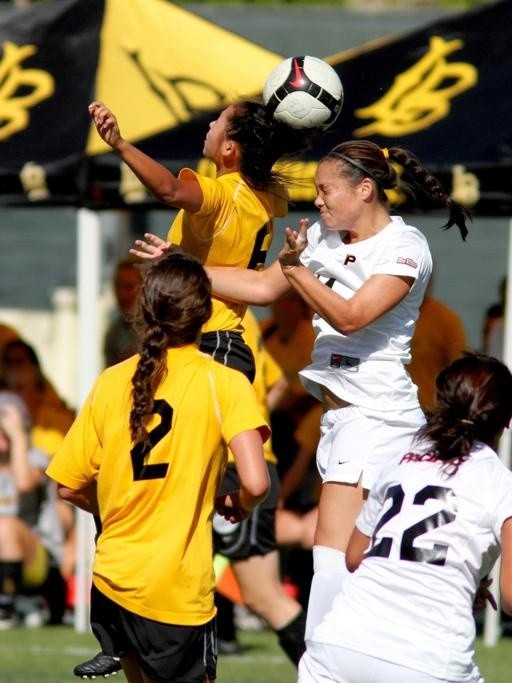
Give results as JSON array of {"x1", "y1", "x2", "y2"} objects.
[{"x1": 264, "y1": 54, "x2": 345, "y2": 135}]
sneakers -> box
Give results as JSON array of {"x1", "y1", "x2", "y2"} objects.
[{"x1": 72, "y1": 652, "x2": 122, "y2": 680}]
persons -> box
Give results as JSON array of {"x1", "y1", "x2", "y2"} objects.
[
  {"x1": 209, "y1": 301, "x2": 307, "y2": 672},
  {"x1": 2, "y1": 248, "x2": 510, "y2": 657},
  {"x1": 127, "y1": 139, "x2": 469, "y2": 669},
  {"x1": 72, "y1": 97, "x2": 289, "y2": 678},
  {"x1": 42, "y1": 251, "x2": 275, "y2": 683},
  {"x1": 290, "y1": 351, "x2": 512, "y2": 683}
]
